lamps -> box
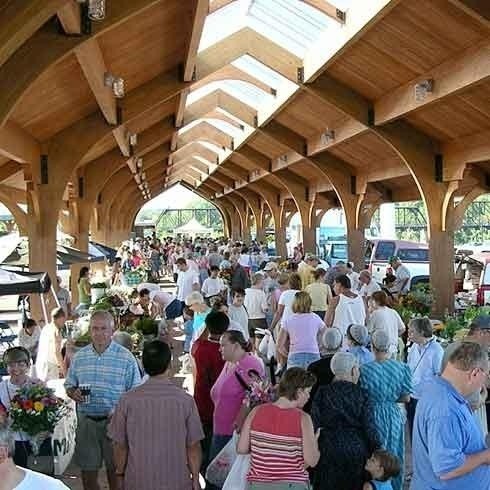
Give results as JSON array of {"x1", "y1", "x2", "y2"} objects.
[
  {"x1": 127, "y1": 132, "x2": 137, "y2": 147},
  {"x1": 135, "y1": 156, "x2": 152, "y2": 203},
  {"x1": 222, "y1": 166, "x2": 263, "y2": 197},
  {"x1": 103, "y1": 69, "x2": 127, "y2": 101},
  {"x1": 79, "y1": 0, "x2": 109, "y2": 37},
  {"x1": 408, "y1": 75, "x2": 438, "y2": 106},
  {"x1": 276, "y1": 154, "x2": 287, "y2": 167},
  {"x1": 319, "y1": 127, "x2": 336, "y2": 146}
]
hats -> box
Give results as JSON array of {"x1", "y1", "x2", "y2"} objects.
[
  {"x1": 310, "y1": 255, "x2": 322, "y2": 265},
  {"x1": 264, "y1": 263, "x2": 278, "y2": 272},
  {"x1": 317, "y1": 324, "x2": 396, "y2": 355}
]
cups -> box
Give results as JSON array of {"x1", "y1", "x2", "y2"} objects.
[{"x1": 78, "y1": 383, "x2": 92, "y2": 404}]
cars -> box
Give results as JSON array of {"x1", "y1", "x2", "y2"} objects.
[{"x1": 259, "y1": 219, "x2": 490, "y2": 310}]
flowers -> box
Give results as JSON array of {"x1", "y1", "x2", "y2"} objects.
[
  {"x1": 6, "y1": 380, "x2": 74, "y2": 456},
  {"x1": 240, "y1": 377, "x2": 278, "y2": 409}
]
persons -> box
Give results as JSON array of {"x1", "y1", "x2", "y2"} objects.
[{"x1": 1, "y1": 231, "x2": 490, "y2": 490}]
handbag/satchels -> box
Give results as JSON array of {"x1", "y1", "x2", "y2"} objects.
[{"x1": 27, "y1": 455, "x2": 54, "y2": 475}]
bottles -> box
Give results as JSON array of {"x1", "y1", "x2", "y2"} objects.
[
  {"x1": 71, "y1": 329, "x2": 79, "y2": 340},
  {"x1": 64, "y1": 320, "x2": 72, "y2": 337}
]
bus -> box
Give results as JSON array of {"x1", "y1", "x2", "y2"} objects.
[{"x1": 135, "y1": 223, "x2": 157, "y2": 241}]
